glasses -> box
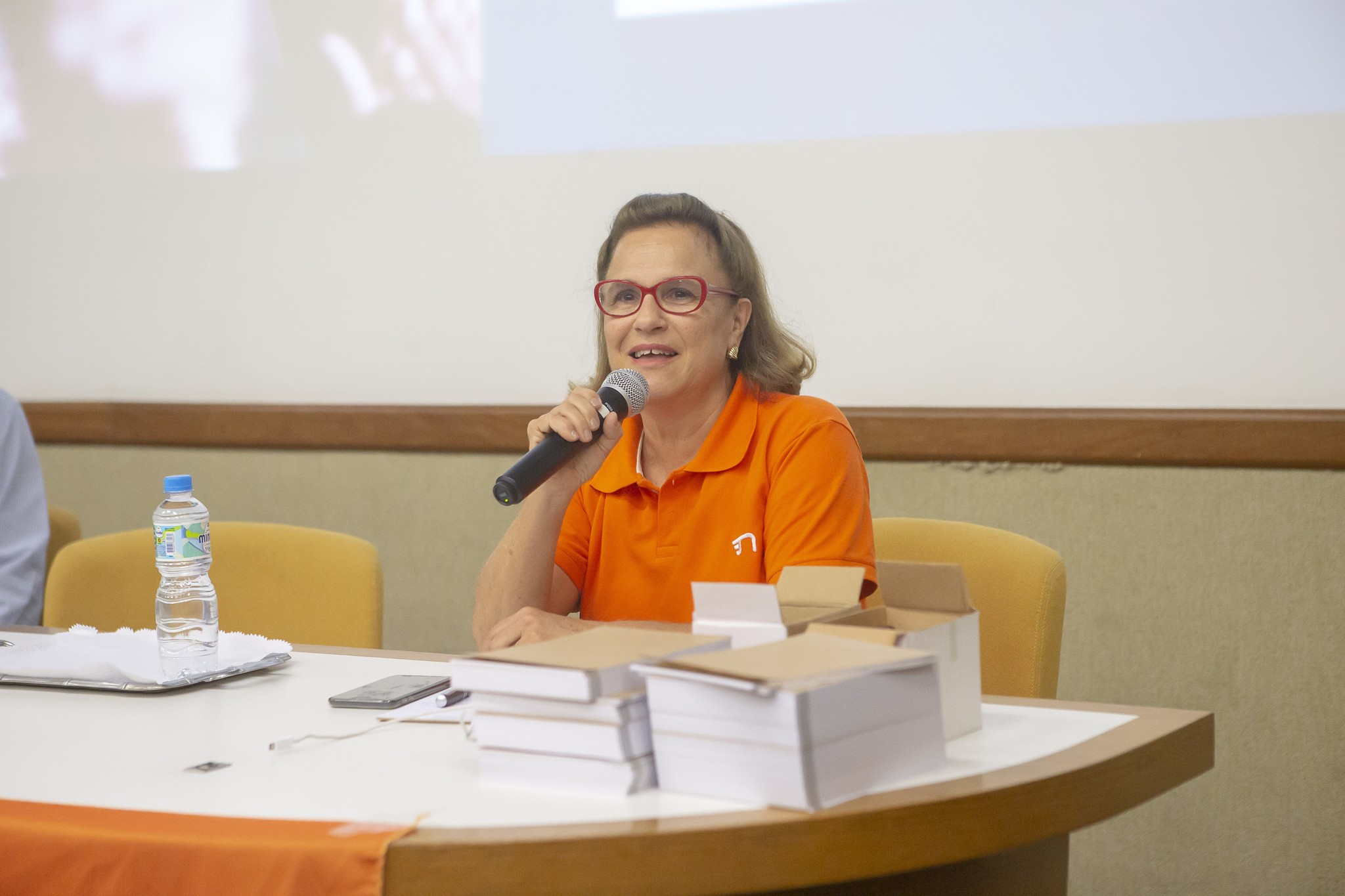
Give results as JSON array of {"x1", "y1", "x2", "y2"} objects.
[{"x1": 594, "y1": 276, "x2": 737, "y2": 318}]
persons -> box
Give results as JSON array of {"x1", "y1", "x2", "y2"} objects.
[
  {"x1": 0, "y1": 388, "x2": 50, "y2": 629},
  {"x1": 466, "y1": 193, "x2": 876, "y2": 654}
]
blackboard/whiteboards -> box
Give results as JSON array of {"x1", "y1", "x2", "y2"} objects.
[{"x1": 0, "y1": 3, "x2": 1344, "y2": 466}]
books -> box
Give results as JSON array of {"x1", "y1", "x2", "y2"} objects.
[{"x1": 449, "y1": 624, "x2": 949, "y2": 815}]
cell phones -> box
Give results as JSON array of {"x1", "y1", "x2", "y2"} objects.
[{"x1": 330, "y1": 676, "x2": 451, "y2": 710}]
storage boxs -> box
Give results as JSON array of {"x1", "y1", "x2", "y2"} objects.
[
  {"x1": 690, "y1": 565, "x2": 868, "y2": 652},
  {"x1": 803, "y1": 557, "x2": 983, "y2": 741}
]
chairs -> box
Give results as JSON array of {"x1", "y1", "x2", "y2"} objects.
[
  {"x1": 869, "y1": 516, "x2": 1065, "y2": 698},
  {"x1": 35, "y1": 507, "x2": 384, "y2": 651}
]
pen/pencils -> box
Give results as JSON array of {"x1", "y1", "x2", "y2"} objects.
[
  {"x1": 436, "y1": 687, "x2": 471, "y2": 708},
  {"x1": 0, "y1": 639, "x2": 14, "y2": 647}
]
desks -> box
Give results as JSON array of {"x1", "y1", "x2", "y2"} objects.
[{"x1": 2, "y1": 617, "x2": 1218, "y2": 896}]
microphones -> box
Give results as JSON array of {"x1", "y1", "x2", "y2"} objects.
[{"x1": 491, "y1": 369, "x2": 650, "y2": 507}]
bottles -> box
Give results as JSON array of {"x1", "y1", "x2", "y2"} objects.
[{"x1": 153, "y1": 474, "x2": 218, "y2": 655}]
